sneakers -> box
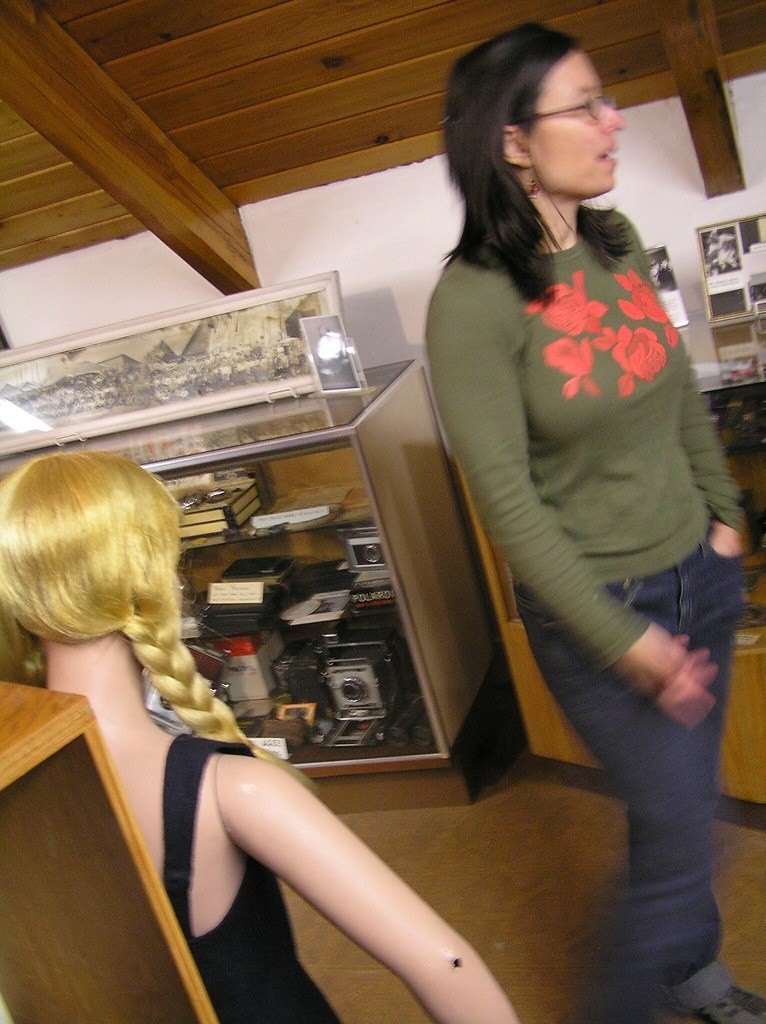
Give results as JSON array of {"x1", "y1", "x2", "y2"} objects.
[{"x1": 696, "y1": 985, "x2": 766, "y2": 1024}]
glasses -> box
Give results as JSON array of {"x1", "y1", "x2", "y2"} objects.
[{"x1": 509, "y1": 96, "x2": 617, "y2": 126}]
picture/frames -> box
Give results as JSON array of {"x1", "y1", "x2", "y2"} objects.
[
  {"x1": 696, "y1": 212, "x2": 766, "y2": 326},
  {"x1": 0, "y1": 270, "x2": 346, "y2": 455},
  {"x1": 297, "y1": 314, "x2": 375, "y2": 400}
]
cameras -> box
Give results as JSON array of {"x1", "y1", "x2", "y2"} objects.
[
  {"x1": 321, "y1": 612, "x2": 412, "y2": 720},
  {"x1": 347, "y1": 534, "x2": 389, "y2": 573}
]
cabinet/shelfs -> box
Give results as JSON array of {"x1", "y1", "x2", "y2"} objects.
[
  {"x1": 451, "y1": 322, "x2": 766, "y2": 814},
  {"x1": 0, "y1": 680, "x2": 221, "y2": 1023},
  {"x1": 147, "y1": 353, "x2": 497, "y2": 805}
]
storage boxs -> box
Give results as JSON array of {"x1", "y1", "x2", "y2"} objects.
[
  {"x1": 244, "y1": 702, "x2": 316, "y2": 760},
  {"x1": 205, "y1": 625, "x2": 284, "y2": 702}
]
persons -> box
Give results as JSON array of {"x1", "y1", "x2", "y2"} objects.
[
  {"x1": 422, "y1": 22, "x2": 766, "y2": 1024},
  {"x1": 317, "y1": 325, "x2": 352, "y2": 375},
  {"x1": 0, "y1": 447, "x2": 518, "y2": 1023}
]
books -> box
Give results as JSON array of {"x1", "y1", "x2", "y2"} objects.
[{"x1": 142, "y1": 480, "x2": 353, "y2": 749}]
